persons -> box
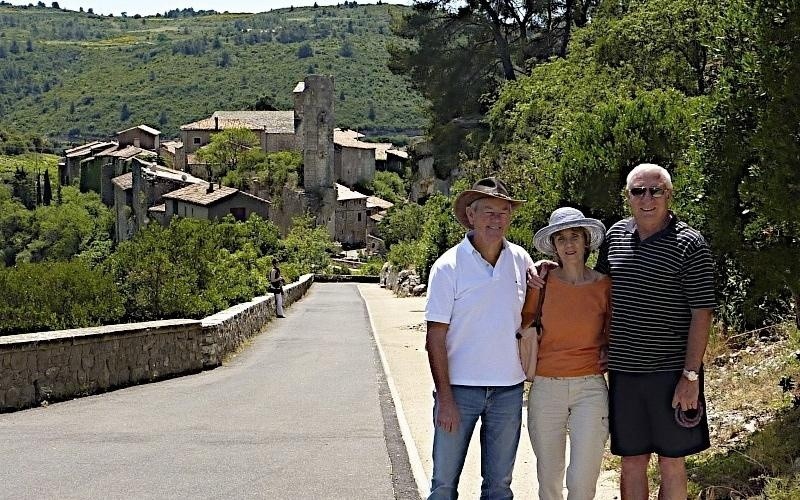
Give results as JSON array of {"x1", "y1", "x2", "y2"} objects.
[
  {"x1": 268, "y1": 256, "x2": 289, "y2": 319},
  {"x1": 423, "y1": 174, "x2": 537, "y2": 500},
  {"x1": 520, "y1": 206, "x2": 611, "y2": 500},
  {"x1": 526, "y1": 162, "x2": 717, "y2": 499}
]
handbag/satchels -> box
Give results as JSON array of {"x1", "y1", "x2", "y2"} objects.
[{"x1": 514, "y1": 326, "x2": 543, "y2": 382}]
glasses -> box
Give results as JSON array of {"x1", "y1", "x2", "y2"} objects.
[{"x1": 628, "y1": 187, "x2": 670, "y2": 198}]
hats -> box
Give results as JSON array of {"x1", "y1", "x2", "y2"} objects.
[
  {"x1": 532, "y1": 206, "x2": 607, "y2": 257},
  {"x1": 454, "y1": 177, "x2": 527, "y2": 232}
]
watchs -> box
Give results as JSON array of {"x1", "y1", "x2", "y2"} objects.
[{"x1": 683, "y1": 368, "x2": 701, "y2": 383}]
